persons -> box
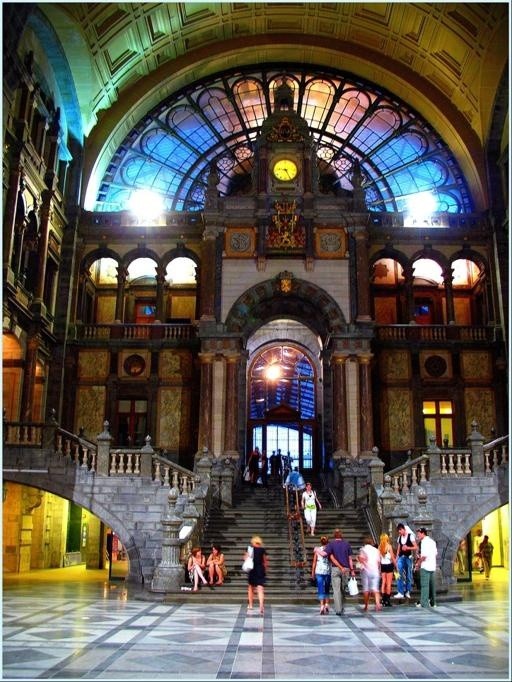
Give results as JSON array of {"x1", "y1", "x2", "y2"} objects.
[
  {"x1": 241, "y1": 534, "x2": 269, "y2": 614},
  {"x1": 301, "y1": 483, "x2": 323, "y2": 536},
  {"x1": 473, "y1": 528, "x2": 485, "y2": 572},
  {"x1": 456, "y1": 538, "x2": 467, "y2": 574},
  {"x1": 187, "y1": 546, "x2": 208, "y2": 592},
  {"x1": 206, "y1": 543, "x2": 229, "y2": 586},
  {"x1": 311, "y1": 522, "x2": 439, "y2": 615},
  {"x1": 247, "y1": 446, "x2": 306, "y2": 511},
  {"x1": 478, "y1": 534, "x2": 494, "y2": 581}
]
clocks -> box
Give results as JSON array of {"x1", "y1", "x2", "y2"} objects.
[{"x1": 272, "y1": 158, "x2": 298, "y2": 183}]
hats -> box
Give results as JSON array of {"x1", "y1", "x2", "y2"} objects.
[{"x1": 250, "y1": 535, "x2": 263, "y2": 544}]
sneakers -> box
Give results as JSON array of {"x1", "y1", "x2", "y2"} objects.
[
  {"x1": 246, "y1": 602, "x2": 265, "y2": 617},
  {"x1": 305, "y1": 528, "x2": 316, "y2": 537},
  {"x1": 193, "y1": 577, "x2": 223, "y2": 592},
  {"x1": 319, "y1": 590, "x2": 437, "y2": 616},
  {"x1": 485, "y1": 575, "x2": 489, "y2": 578}
]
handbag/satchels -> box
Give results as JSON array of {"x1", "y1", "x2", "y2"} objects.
[
  {"x1": 304, "y1": 504, "x2": 316, "y2": 510},
  {"x1": 393, "y1": 570, "x2": 401, "y2": 580},
  {"x1": 348, "y1": 576, "x2": 359, "y2": 596},
  {"x1": 243, "y1": 466, "x2": 251, "y2": 482}
]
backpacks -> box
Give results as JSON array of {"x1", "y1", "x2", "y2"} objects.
[{"x1": 241, "y1": 545, "x2": 256, "y2": 573}]
